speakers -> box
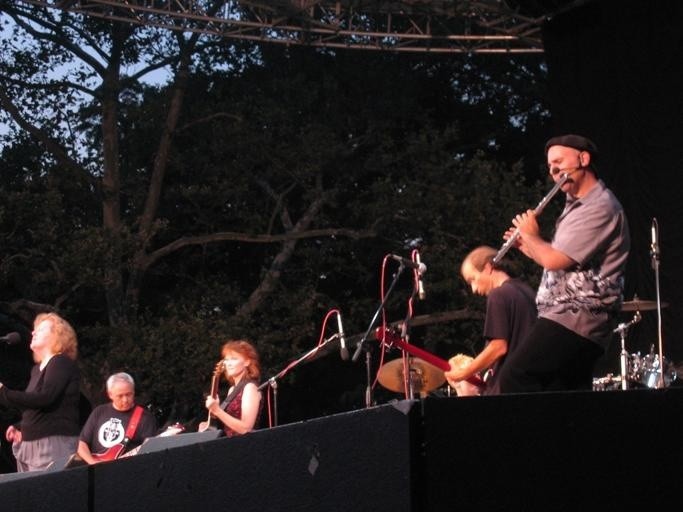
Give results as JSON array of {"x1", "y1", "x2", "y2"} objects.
[{"x1": 137, "y1": 429, "x2": 223, "y2": 456}]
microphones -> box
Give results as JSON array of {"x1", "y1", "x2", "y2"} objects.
[
  {"x1": 650, "y1": 224, "x2": 662, "y2": 269},
  {"x1": 335, "y1": 310, "x2": 352, "y2": 362},
  {"x1": 552, "y1": 167, "x2": 582, "y2": 174},
  {"x1": 414, "y1": 249, "x2": 428, "y2": 301}
]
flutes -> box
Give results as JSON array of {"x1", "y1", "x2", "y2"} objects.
[{"x1": 493, "y1": 172, "x2": 568, "y2": 265}]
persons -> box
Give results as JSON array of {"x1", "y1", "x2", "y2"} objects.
[
  {"x1": 0, "y1": 311, "x2": 81, "y2": 473},
  {"x1": 74, "y1": 371, "x2": 160, "y2": 465},
  {"x1": 487, "y1": 135, "x2": 632, "y2": 392},
  {"x1": 197, "y1": 337, "x2": 264, "y2": 437},
  {"x1": 444, "y1": 245, "x2": 537, "y2": 396}
]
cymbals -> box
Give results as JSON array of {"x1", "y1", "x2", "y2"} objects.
[
  {"x1": 377, "y1": 356, "x2": 447, "y2": 394},
  {"x1": 619, "y1": 300, "x2": 667, "y2": 311}
]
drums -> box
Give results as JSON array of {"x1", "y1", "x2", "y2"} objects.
[
  {"x1": 633, "y1": 352, "x2": 676, "y2": 389},
  {"x1": 592, "y1": 376, "x2": 629, "y2": 391}
]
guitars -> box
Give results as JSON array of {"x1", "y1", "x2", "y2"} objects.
[
  {"x1": 198, "y1": 359, "x2": 225, "y2": 433},
  {"x1": 82, "y1": 423, "x2": 186, "y2": 465},
  {"x1": 376, "y1": 323, "x2": 494, "y2": 396}
]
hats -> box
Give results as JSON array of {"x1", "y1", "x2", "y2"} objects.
[{"x1": 544, "y1": 135, "x2": 602, "y2": 167}]
